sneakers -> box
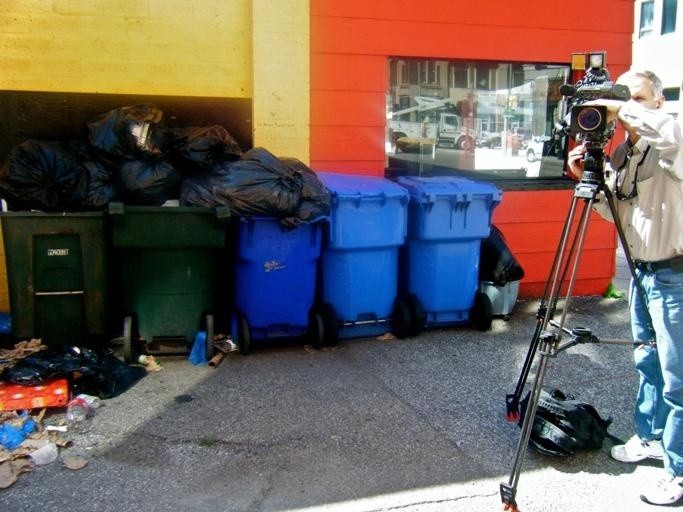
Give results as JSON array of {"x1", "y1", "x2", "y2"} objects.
[
  {"x1": 610, "y1": 434, "x2": 664, "y2": 463},
  {"x1": 639, "y1": 473, "x2": 682, "y2": 505}
]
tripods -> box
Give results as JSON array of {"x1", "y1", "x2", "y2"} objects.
[{"x1": 499, "y1": 182, "x2": 656, "y2": 512}]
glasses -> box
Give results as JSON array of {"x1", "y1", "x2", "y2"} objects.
[{"x1": 615, "y1": 168, "x2": 637, "y2": 201}]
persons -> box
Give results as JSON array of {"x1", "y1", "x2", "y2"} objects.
[{"x1": 581, "y1": 69, "x2": 682, "y2": 505}]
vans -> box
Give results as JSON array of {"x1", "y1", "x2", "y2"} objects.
[{"x1": 510, "y1": 127, "x2": 533, "y2": 149}]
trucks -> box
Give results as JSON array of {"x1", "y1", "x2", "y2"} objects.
[{"x1": 388, "y1": 112, "x2": 475, "y2": 152}]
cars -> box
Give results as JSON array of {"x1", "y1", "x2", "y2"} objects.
[
  {"x1": 525, "y1": 136, "x2": 552, "y2": 162},
  {"x1": 477, "y1": 132, "x2": 501, "y2": 148}
]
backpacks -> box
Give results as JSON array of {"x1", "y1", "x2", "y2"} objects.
[{"x1": 517, "y1": 387, "x2": 625, "y2": 459}]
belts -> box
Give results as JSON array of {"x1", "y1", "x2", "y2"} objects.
[{"x1": 635, "y1": 257, "x2": 682, "y2": 271}]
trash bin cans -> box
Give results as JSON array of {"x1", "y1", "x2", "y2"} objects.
[
  {"x1": 1, "y1": 211, "x2": 110, "y2": 346},
  {"x1": 314, "y1": 170, "x2": 412, "y2": 350},
  {"x1": 234, "y1": 215, "x2": 327, "y2": 355},
  {"x1": 397, "y1": 175, "x2": 503, "y2": 338},
  {"x1": 110, "y1": 204, "x2": 232, "y2": 365}
]
